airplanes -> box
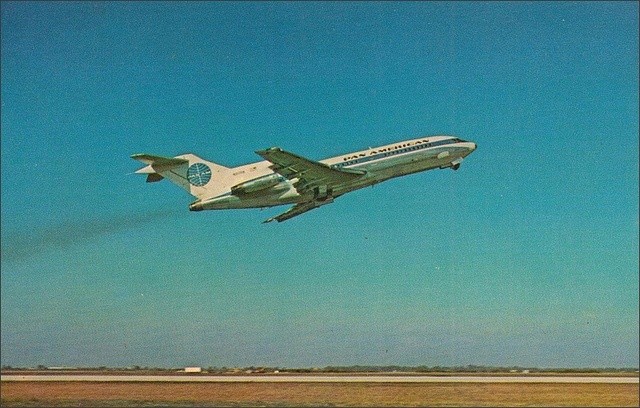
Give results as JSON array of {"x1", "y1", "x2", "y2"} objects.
[{"x1": 124, "y1": 136, "x2": 479, "y2": 224}]
